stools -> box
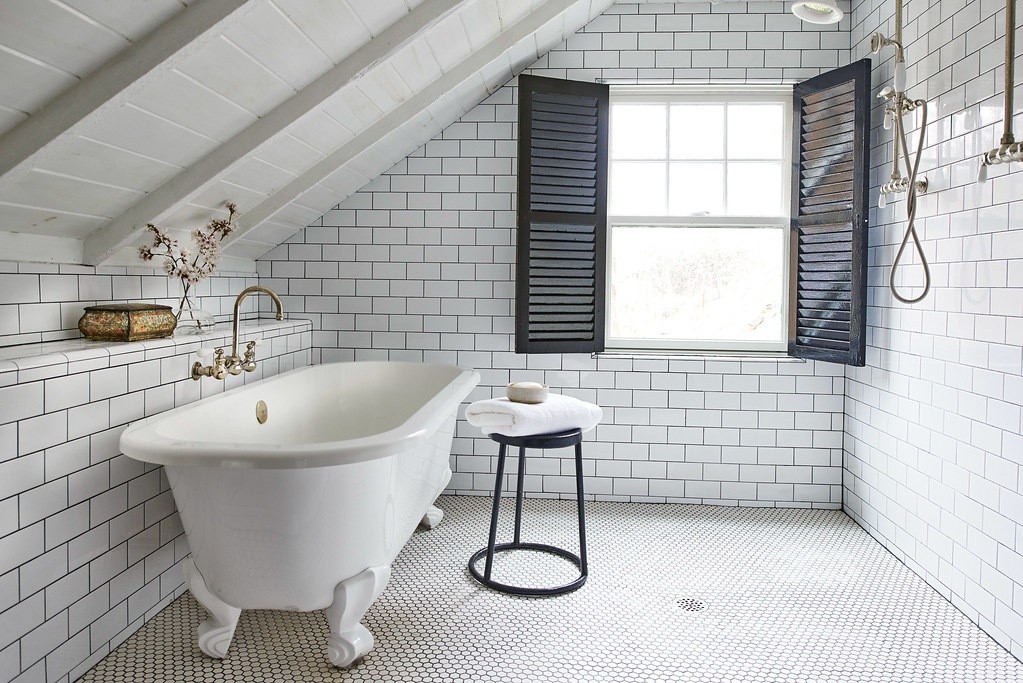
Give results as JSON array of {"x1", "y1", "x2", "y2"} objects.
[{"x1": 469, "y1": 428, "x2": 588, "y2": 595}]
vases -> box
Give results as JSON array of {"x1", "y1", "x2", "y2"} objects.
[{"x1": 172, "y1": 280, "x2": 216, "y2": 336}]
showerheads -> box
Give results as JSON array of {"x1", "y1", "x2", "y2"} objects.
[{"x1": 869, "y1": 33, "x2": 908, "y2": 95}]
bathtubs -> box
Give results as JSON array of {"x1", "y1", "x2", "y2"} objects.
[{"x1": 120, "y1": 360, "x2": 481, "y2": 666}]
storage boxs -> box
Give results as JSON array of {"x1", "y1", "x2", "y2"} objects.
[{"x1": 78, "y1": 304, "x2": 177, "y2": 341}]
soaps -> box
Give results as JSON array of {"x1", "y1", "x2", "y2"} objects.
[{"x1": 511, "y1": 381, "x2": 543, "y2": 390}]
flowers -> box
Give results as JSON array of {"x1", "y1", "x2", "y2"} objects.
[{"x1": 137, "y1": 200, "x2": 241, "y2": 330}]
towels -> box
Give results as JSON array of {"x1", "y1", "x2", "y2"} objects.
[{"x1": 466, "y1": 391, "x2": 603, "y2": 437}]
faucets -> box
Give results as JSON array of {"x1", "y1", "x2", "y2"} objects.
[{"x1": 220, "y1": 286, "x2": 285, "y2": 374}]
